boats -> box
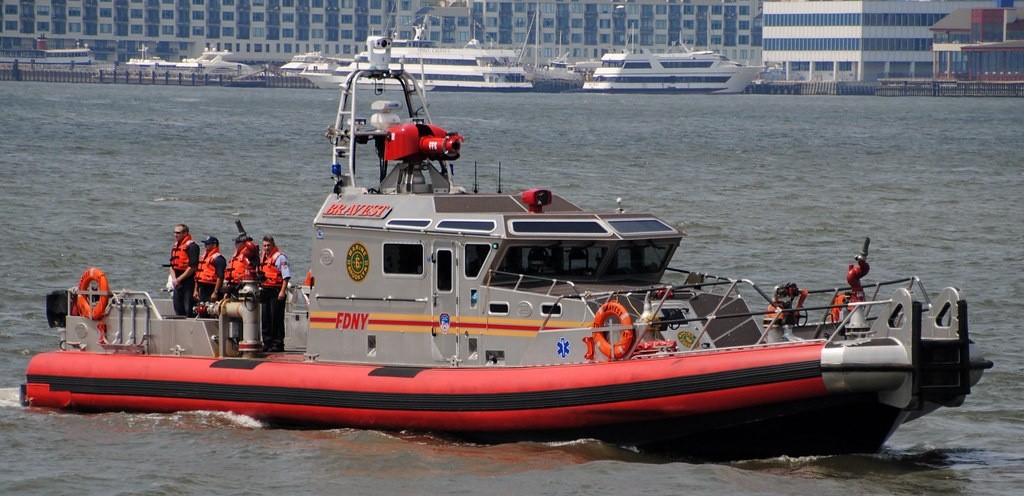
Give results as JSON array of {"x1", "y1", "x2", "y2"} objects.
[
  {"x1": 298, "y1": 15, "x2": 534, "y2": 93},
  {"x1": 280, "y1": 51, "x2": 355, "y2": 74},
  {"x1": 581, "y1": 50, "x2": 767, "y2": 94},
  {"x1": 17, "y1": 34, "x2": 993, "y2": 455},
  {"x1": 176, "y1": 48, "x2": 252, "y2": 70},
  {"x1": 1, "y1": 43, "x2": 93, "y2": 65},
  {"x1": 126, "y1": 43, "x2": 177, "y2": 67}
]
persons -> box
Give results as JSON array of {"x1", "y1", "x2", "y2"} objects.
[{"x1": 169, "y1": 224, "x2": 292, "y2": 352}]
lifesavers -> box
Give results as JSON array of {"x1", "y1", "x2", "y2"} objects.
[
  {"x1": 74, "y1": 266, "x2": 111, "y2": 321},
  {"x1": 590, "y1": 301, "x2": 634, "y2": 358},
  {"x1": 828, "y1": 294, "x2": 851, "y2": 322}
]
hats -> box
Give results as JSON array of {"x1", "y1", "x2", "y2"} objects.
[
  {"x1": 201, "y1": 236, "x2": 219, "y2": 247},
  {"x1": 232, "y1": 235, "x2": 246, "y2": 243}
]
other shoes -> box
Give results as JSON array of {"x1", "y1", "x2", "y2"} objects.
[{"x1": 262, "y1": 344, "x2": 284, "y2": 353}]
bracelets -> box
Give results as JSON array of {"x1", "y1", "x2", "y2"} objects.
[{"x1": 215, "y1": 291, "x2": 218, "y2": 294}]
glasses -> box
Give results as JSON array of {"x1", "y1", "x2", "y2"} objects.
[{"x1": 174, "y1": 231, "x2": 181, "y2": 234}]
